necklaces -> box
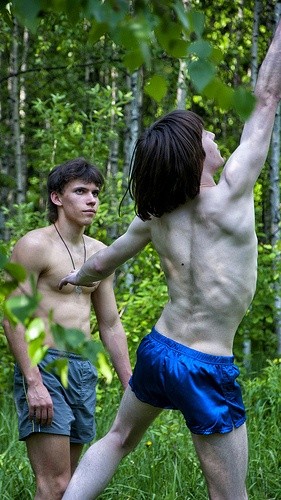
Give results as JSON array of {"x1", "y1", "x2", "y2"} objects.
[{"x1": 52, "y1": 222, "x2": 87, "y2": 296}]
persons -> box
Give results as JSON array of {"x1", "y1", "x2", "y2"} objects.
[
  {"x1": 59, "y1": 17, "x2": 281, "y2": 500},
  {"x1": 1, "y1": 158, "x2": 133, "y2": 500}
]
